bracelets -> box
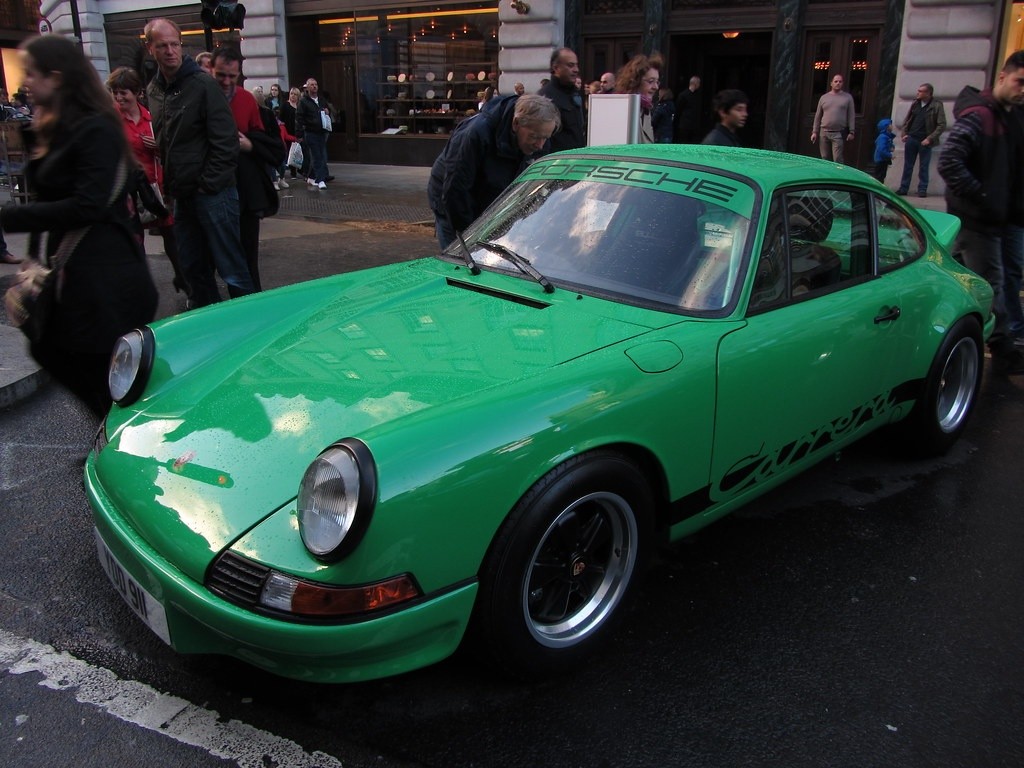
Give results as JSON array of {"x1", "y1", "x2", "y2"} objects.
[{"x1": 850, "y1": 132, "x2": 854, "y2": 135}]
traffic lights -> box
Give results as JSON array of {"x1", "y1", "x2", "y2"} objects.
[{"x1": 201, "y1": 0, "x2": 246, "y2": 29}]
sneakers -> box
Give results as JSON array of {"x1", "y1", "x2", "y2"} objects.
[
  {"x1": 273, "y1": 181, "x2": 280, "y2": 191},
  {"x1": 318, "y1": 181, "x2": 327, "y2": 188},
  {"x1": 278, "y1": 178, "x2": 289, "y2": 188},
  {"x1": 307, "y1": 178, "x2": 318, "y2": 185}
]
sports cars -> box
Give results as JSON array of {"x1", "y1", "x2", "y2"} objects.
[{"x1": 84, "y1": 144, "x2": 996, "y2": 685}]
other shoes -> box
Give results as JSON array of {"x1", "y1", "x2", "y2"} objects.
[
  {"x1": 187, "y1": 299, "x2": 195, "y2": 309},
  {"x1": 290, "y1": 173, "x2": 297, "y2": 179},
  {"x1": 895, "y1": 190, "x2": 907, "y2": 195},
  {"x1": 325, "y1": 176, "x2": 335, "y2": 181},
  {"x1": 918, "y1": 190, "x2": 927, "y2": 196},
  {"x1": 987, "y1": 341, "x2": 1024, "y2": 374}
]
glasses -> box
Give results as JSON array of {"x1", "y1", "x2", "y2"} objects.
[{"x1": 641, "y1": 78, "x2": 661, "y2": 86}]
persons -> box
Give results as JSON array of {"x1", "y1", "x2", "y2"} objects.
[
  {"x1": 612, "y1": 54, "x2": 663, "y2": 144},
  {"x1": 0, "y1": 36, "x2": 160, "y2": 421},
  {"x1": 895, "y1": 83, "x2": 946, "y2": 198},
  {"x1": 937, "y1": 50, "x2": 1024, "y2": 375},
  {"x1": 700, "y1": 89, "x2": 750, "y2": 146},
  {"x1": 478, "y1": 73, "x2": 700, "y2": 143},
  {"x1": 874, "y1": 119, "x2": 896, "y2": 184},
  {"x1": 144, "y1": 17, "x2": 253, "y2": 308},
  {"x1": 195, "y1": 51, "x2": 212, "y2": 73},
  {"x1": 427, "y1": 95, "x2": 561, "y2": 250},
  {"x1": 811, "y1": 74, "x2": 855, "y2": 164},
  {"x1": 0, "y1": 65, "x2": 335, "y2": 298},
  {"x1": 209, "y1": 47, "x2": 285, "y2": 299},
  {"x1": 534, "y1": 48, "x2": 588, "y2": 159}
]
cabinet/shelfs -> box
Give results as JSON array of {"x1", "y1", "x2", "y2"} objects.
[{"x1": 374, "y1": 60, "x2": 498, "y2": 134}]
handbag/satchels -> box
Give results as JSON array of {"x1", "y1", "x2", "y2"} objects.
[
  {"x1": 4, "y1": 258, "x2": 65, "y2": 337},
  {"x1": 136, "y1": 183, "x2": 165, "y2": 223},
  {"x1": 287, "y1": 141, "x2": 304, "y2": 170},
  {"x1": 321, "y1": 110, "x2": 332, "y2": 131}
]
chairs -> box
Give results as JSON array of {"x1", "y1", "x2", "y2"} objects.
[
  {"x1": 773, "y1": 192, "x2": 843, "y2": 291},
  {"x1": 599, "y1": 189, "x2": 707, "y2": 295}
]
